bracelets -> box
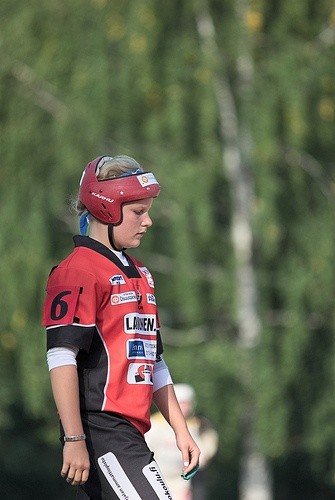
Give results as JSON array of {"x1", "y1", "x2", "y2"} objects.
[{"x1": 64, "y1": 434, "x2": 86, "y2": 442}]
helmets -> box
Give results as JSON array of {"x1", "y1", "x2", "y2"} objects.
[{"x1": 79, "y1": 156, "x2": 162, "y2": 226}]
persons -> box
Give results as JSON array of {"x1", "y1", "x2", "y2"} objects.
[
  {"x1": 42, "y1": 155, "x2": 201, "y2": 500},
  {"x1": 144, "y1": 383, "x2": 219, "y2": 500}
]
